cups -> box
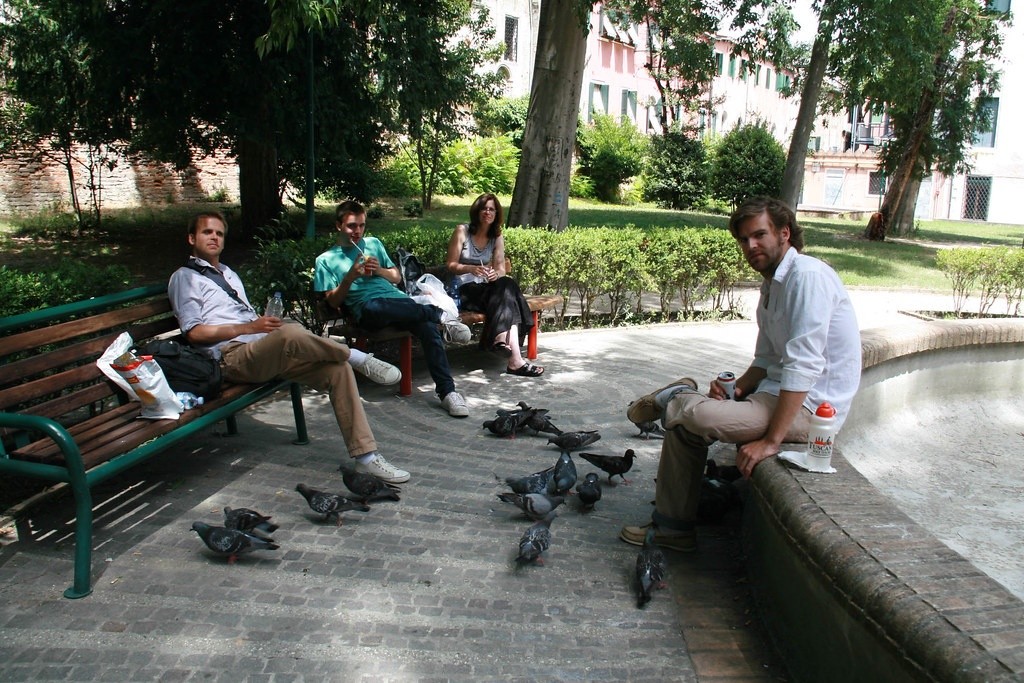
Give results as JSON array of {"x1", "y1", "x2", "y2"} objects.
[
  {"x1": 362, "y1": 255, "x2": 373, "y2": 275},
  {"x1": 480, "y1": 266, "x2": 492, "y2": 283}
]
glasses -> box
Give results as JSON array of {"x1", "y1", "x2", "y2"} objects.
[{"x1": 480, "y1": 207, "x2": 497, "y2": 216}]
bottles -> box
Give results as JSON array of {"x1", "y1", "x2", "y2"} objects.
[
  {"x1": 173, "y1": 391, "x2": 203, "y2": 411},
  {"x1": 807, "y1": 402, "x2": 837, "y2": 469},
  {"x1": 265, "y1": 291, "x2": 283, "y2": 322}
]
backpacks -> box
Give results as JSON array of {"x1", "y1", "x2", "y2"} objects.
[{"x1": 129, "y1": 339, "x2": 224, "y2": 400}]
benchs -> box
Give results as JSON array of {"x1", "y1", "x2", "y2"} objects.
[
  {"x1": 0, "y1": 279, "x2": 312, "y2": 600},
  {"x1": 328, "y1": 292, "x2": 565, "y2": 398}
]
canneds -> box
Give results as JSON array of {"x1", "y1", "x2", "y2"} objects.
[
  {"x1": 716, "y1": 372, "x2": 736, "y2": 400},
  {"x1": 363, "y1": 257, "x2": 373, "y2": 278}
]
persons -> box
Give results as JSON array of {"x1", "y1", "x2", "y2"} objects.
[
  {"x1": 447, "y1": 193, "x2": 544, "y2": 377},
  {"x1": 168, "y1": 211, "x2": 410, "y2": 483},
  {"x1": 314, "y1": 201, "x2": 470, "y2": 416},
  {"x1": 621, "y1": 193, "x2": 861, "y2": 552}
]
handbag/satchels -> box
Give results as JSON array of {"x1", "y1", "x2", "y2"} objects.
[
  {"x1": 409, "y1": 273, "x2": 459, "y2": 324},
  {"x1": 94, "y1": 333, "x2": 184, "y2": 419}
]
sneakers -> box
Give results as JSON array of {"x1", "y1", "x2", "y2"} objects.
[
  {"x1": 353, "y1": 352, "x2": 403, "y2": 385},
  {"x1": 353, "y1": 453, "x2": 410, "y2": 483},
  {"x1": 620, "y1": 517, "x2": 697, "y2": 552},
  {"x1": 441, "y1": 391, "x2": 469, "y2": 416},
  {"x1": 627, "y1": 376, "x2": 699, "y2": 423},
  {"x1": 438, "y1": 310, "x2": 471, "y2": 343}
]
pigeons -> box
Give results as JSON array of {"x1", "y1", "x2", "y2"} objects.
[
  {"x1": 502, "y1": 469, "x2": 555, "y2": 495},
  {"x1": 190, "y1": 522, "x2": 279, "y2": 564},
  {"x1": 555, "y1": 447, "x2": 577, "y2": 493},
  {"x1": 482, "y1": 402, "x2": 562, "y2": 438},
  {"x1": 579, "y1": 450, "x2": 637, "y2": 485},
  {"x1": 337, "y1": 462, "x2": 401, "y2": 505},
  {"x1": 514, "y1": 511, "x2": 559, "y2": 563},
  {"x1": 576, "y1": 472, "x2": 602, "y2": 513},
  {"x1": 296, "y1": 484, "x2": 371, "y2": 525},
  {"x1": 547, "y1": 429, "x2": 600, "y2": 451},
  {"x1": 223, "y1": 506, "x2": 278, "y2": 542},
  {"x1": 628, "y1": 401, "x2": 665, "y2": 441},
  {"x1": 635, "y1": 528, "x2": 666, "y2": 601},
  {"x1": 496, "y1": 492, "x2": 566, "y2": 521}
]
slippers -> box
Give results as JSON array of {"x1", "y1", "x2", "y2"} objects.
[
  {"x1": 507, "y1": 362, "x2": 544, "y2": 377},
  {"x1": 490, "y1": 341, "x2": 513, "y2": 358}
]
